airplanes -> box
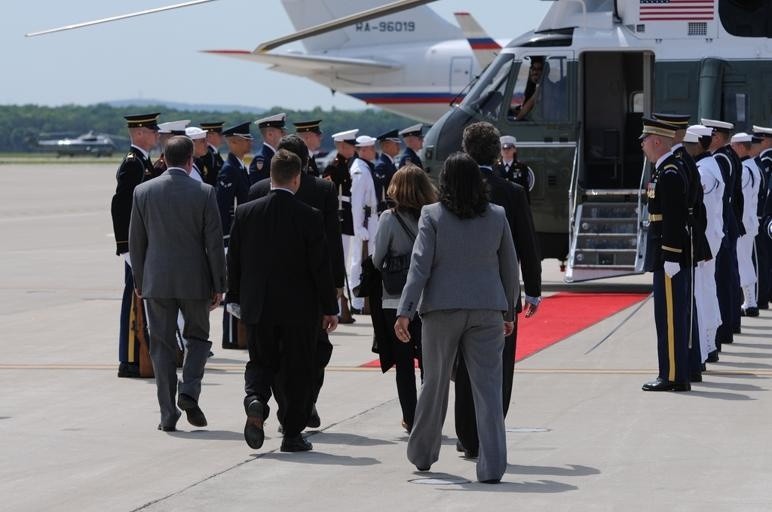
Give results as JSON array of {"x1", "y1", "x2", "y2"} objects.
[
  {"x1": 34, "y1": 128, "x2": 131, "y2": 158},
  {"x1": 196, "y1": 1, "x2": 514, "y2": 127},
  {"x1": 20, "y1": 1, "x2": 770, "y2": 287}
]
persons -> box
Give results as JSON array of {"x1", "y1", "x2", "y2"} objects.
[
  {"x1": 371, "y1": 122, "x2": 540, "y2": 484},
  {"x1": 228, "y1": 132, "x2": 338, "y2": 453},
  {"x1": 109, "y1": 110, "x2": 340, "y2": 378},
  {"x1": 514, "y1": 61, "x2": 556, "y2": 122},
  {"x1": 324, "y1": 124, "x2": 425, "y2": 324},
  {"x1": 128, "y1": 137, "x2": 226, "y2": 433},
  {"x1": 639, "y1": 111, "x2": 772, "y2": 392}
]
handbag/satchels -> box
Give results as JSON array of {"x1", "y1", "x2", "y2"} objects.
[{"x1": 380, "y1": 266, "x2": 410, "y2": 296}]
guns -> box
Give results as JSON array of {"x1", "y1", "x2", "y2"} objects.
[
  {"x1": 175, "y1": 337, "x2": 184, "y2": 368},
  {"x1": 362, "y1": 205, "x2": 372, "y2": 315},
  {"x1": 381, "y1": 185, "x2": 386, "y2": 213},
  {"x1": 338, "y1": 183, "x2": 351, "y2": 324},
  {"x1": 134, "y1": 292, "x2": 154, "y2": 378},
  {"x1": 233, "y1": 196, "x2": 246, "y2": 349}
]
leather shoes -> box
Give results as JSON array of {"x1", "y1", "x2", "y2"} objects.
[
  {"x1": 720, "y1": 336, "x2": 733, "y2": 344},
  {"x1": 689, "y1": 372, "x2": 703, "y2": 383},
  {"x1": 455, "y1": 440, "x2": 474, "y2": 457},
  {"x1": 221, "y1": 342, "x2": 246, "y2": 350},
  {"x1": 177, "y1": 393, "x2": 208, "y2": 427},
  {"x1": 706, "y1": 350, "x2": 719, "y2": 362},
  {"x1": 741, "y1": 307, "x2": 759, "y2": 318},
  {"x1": 482, "y1": 479, "x2": 502, "y2": 484},
  {"x1": 157, "y1": 423, "x2": 176, "y2": 432},
  {"x1": 280, "y1": 436, "x2": 313, "y2": 452},
  {"x1": 733, "y1": 326, "x2": 741, "y2": 333},
  {"x1": 243, "y1": 398, "x2": 265, "y2": 449},
  {"x1": 338, "y1": 315, "x2": 355, "y2": 324},
  {"x1": 417, "y1": 466, "x2": 431, "y2": 473},
  {"x1": 400, "y1": 419, "x2": 411, "y2": 433},
  {"x1": 641, "y1": 375, "x2": 691, "y2": 392},
  {"x1": 701, "y1": 364, "x2": 707, "y2": 372},
  {"x1": 118, "y1": 362, "x2": 155, "y2": 379},
  {"x1": 309, "y1": 403, "x2": 322, "y2": 429},
  {"x1": 277, "y1": 426, "x2": 286, "y2": 435}
]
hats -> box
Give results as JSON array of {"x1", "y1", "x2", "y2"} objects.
[
  {"x1": 293, "y1": 119, "x2": 323, "y2": 135},
  {"x1": 700, "y1": 118, "x2": 734, "y2": 134},
  {"x1": 332, "y1": 129, "x2": 360, "y2": 145},
  {"x1": 224, "y1": 120, "x2": 256, "y2": 141},
  {"x1": 683, "y1": 131, "x2": 703, "y2": 144},
  {"x1": 500, "y1": 135, "x2": 517, "y2": 150},
  {"x1": 752, "y1": 125, "x2": 772, "y2": 138},
  {"x1": 398, "y1": 123, "x2": 424, "y2": 138},
  {"x1": 255, "y1": 112, "x2": 289, "y2": 131},
  {"x1": 124, "y1": 111, "x2": 161, "y2": 131},
  {"x1": 652, "y1": 112, "x2": 690, "y2": 130},
  {"x1": 637, "y1": 115, "x2": 678, "y2": 141},
  {"x1": 157, "y1": 119, "x2": 192, "y2": 135},
  {"x1": 376, "y1": 128, "x2": 402, "y2": 144},
  {"x1": 729, "y1": 132, "x2": 753, "y2": 144},
  {"x1": 356, "y1": 135, "x2": 377, "y2": 147},
  {"x1": 184, "y1": 127, "x2": 209, "y2": 140},
  {"x1": 750, "y1": 135, "x2": 765, "y2": 143},
  {"x1": 686, "y1": 125, "x2": 714, "y2": 137},
  {"x1": 199, "y1": 120, "x2": 225, "y2": 133}
]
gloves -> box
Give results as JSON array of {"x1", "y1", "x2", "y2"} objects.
[
  {"x1": 662, "y1": 261, "x2": 681, "y2": 278},
  {"x1": 226, "y1": 303, "x2": 242, "y2": 318},
  {"x1": 356, "y1": 227, "x2": 370, "y2": 241},
  {"x1": 123, "y1": 252, "x2": 132, "y2": 269}
]
